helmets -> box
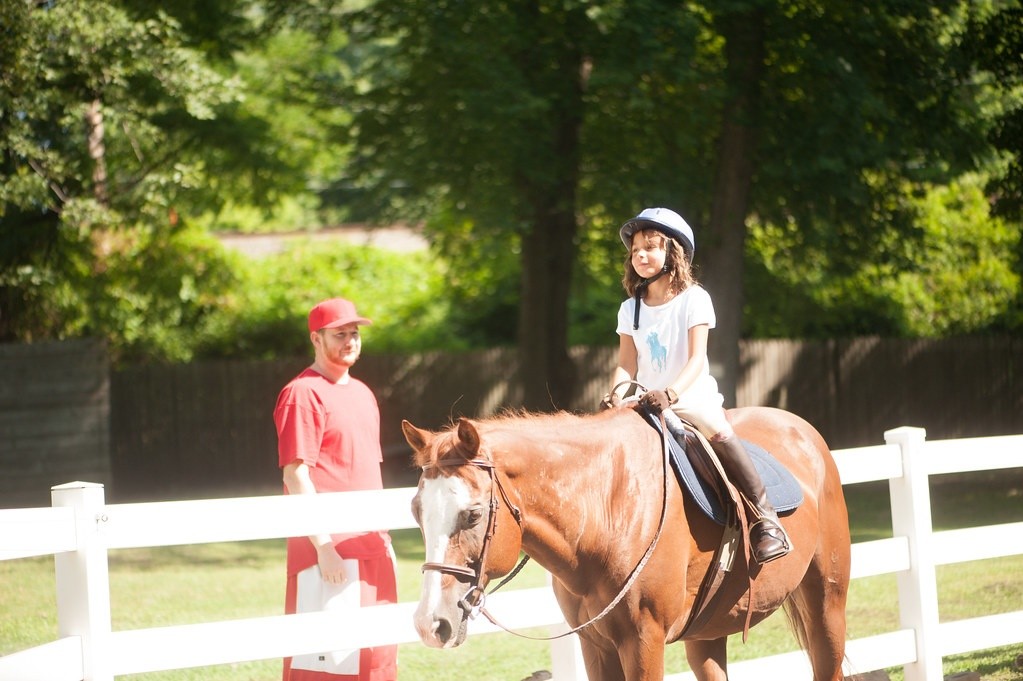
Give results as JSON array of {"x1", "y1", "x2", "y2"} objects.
[{"x1": 619, "y1": 207, "x2": 694, "y2": 267}]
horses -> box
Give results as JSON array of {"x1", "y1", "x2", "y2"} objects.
[{"x1": 402, "y1": 406, "x2": 852, "y2": 681}]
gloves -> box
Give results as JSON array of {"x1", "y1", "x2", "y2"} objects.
[
  {"x1": 599, "y1": 392, "x2": 619, "y2": 411},
  {"x1": 638, "y1": 388, "x2": 679, "y2": 416}
]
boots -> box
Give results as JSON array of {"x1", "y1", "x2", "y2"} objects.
[{"x1": 707, "y1": 432, "x2": 793, "y2": 561}]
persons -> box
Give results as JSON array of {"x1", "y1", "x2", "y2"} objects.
[
  {"x1": 600, "y1": 206, "x2": 793, "y2": 562},
  {"x1": 272, "y1": 299, "x2": 399, "y2": 680}
]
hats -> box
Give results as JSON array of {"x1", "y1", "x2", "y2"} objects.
[{"x1": 308, "y1": 299, "x2": 371, "y2": 332}]
